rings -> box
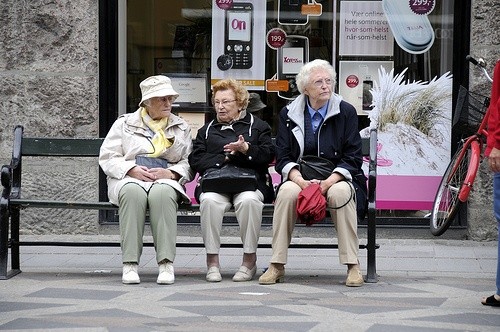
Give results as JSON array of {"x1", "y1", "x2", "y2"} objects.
[{"x1": 492, "y1": 163, "x2": 495, "y2": 165}]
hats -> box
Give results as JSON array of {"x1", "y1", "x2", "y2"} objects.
[{"x1": 137, "y1": 75, "x2": 180, "y2": 108}]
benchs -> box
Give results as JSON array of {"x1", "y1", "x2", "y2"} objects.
[{"x1": 0, "y1": 125, "x2": 381, "y2": 283}]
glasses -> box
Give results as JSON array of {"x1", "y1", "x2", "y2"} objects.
[
  {"x1": 314, "y1": 79, "x2": 333, "y2": 86},
  {"x1": 211, "y1": 98, "x2": 238, "y2": 105}
]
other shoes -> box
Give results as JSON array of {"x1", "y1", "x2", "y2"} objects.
[
  {"x1": 345, "y1": 268, "x2": 364, "y2": 287},
  {"x1": 122, "y1": 263, "x2": 140, "y2": 284},
  {"x1": 206, "y1": 264, "x2": 222, "y2": 282},
  {"x1": 258, "y1": 263, "x2": 286, "y2": 285},
  {"x1": 232, "y1": 264, "x2": 257, "y2": 282},
  {"x1": 156, "y1": 262, "x2": 175, "y2": 285}
]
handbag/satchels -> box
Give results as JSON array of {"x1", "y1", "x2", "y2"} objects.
[
  {"x1": 135, "y1": 155, "x2": 169, "y2": 169},
  {"x1": 199, "y1": 153, "x2": 258, "y2": 193},
  {"x1": 297, "y1": 155, "x2": 337, "y2": 180}
]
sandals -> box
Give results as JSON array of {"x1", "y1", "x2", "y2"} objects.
[{"x1": 481, "y1": 295, "x2": 500, "y2": 308}]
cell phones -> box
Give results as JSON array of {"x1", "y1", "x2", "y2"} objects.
[
  {"x1": 276, "y1": 36, "x2": 309, "y2": 99},
  {"x1": 223, "y1": 2, "x2": 253, "y2": 69}
]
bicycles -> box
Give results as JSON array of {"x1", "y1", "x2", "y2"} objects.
[{"x1": 430, "y1": 55, "x2": 494, "y2": 236}]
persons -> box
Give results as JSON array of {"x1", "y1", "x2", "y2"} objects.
[
  {"x1": 257, "y1": 58, "x2": 372, "y2": 286},
  {"x1": 481, "y1": 60, "x2": 500, "y2": 306},
  {"x1": 189, "y1": 79, "x2": 274, "y2": 282},
  {"x1": 98, "y1": 75, "x2": 197, "y2": 286}
]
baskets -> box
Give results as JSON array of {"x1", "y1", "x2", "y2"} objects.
[{"x1": 452, "y1": 86, "x2": 492, "y2": 146}]
